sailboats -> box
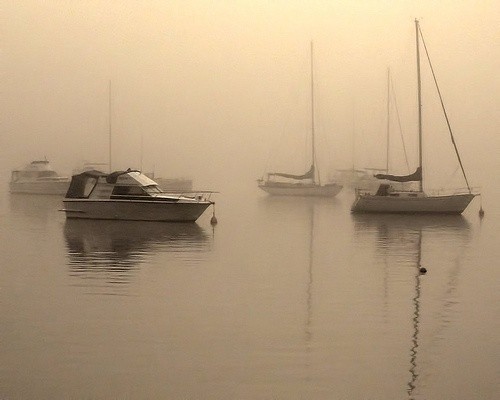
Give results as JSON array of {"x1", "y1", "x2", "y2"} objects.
[
  {"x1": 351, "y1": 17, "x2": 485, "y2": 217},
  {"x1": 9, "y1": 79, "x2": 193, "y2": 194},
  {"x1": 328, "y1": 62, "x2": 412, "y2": 191},
  {"x1": 256, "y1": 41, "x2": 343, "y2": 197}
]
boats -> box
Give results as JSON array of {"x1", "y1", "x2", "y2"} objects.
[{"x1": 57, "y1": 169, "x2": 217, "y2": 227}]
